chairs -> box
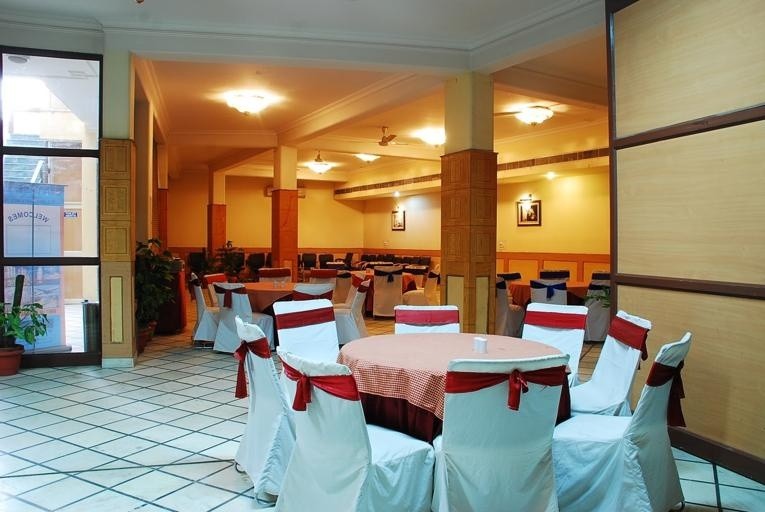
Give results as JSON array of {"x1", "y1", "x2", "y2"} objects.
[
  {"x1": 352, "y1": 353, "x2": 569, "y2": 511},
  {"x1": 496, "y1": 272, "x2": 521, "y2": 281},
  {"x1": 496, "y1": 280, "x2": 525, "y2": 338},
  {"x1": 248, "y1": 252, "x2": 441, "y2": 317},
  {"x1": 274, "y1": 346, "x2": 438, "y2": 509},
  {"x1": 524, "y1": 281, "x2": 565, "y2": 309},
  {"x1": 189, "y1": 273, "x2": 229, "y2": 352},
  {"x1": 539, "y1": 269, "x2": 571, "y2": 281},
  {"x1": 332, "y1": 279, "x2": 371, "y2": 346},
  {"x1": 549, "y1": 328, "x2": 692, "y2": 510},
  {"x1": 211, "y1": 281, "x2": 275, "y2": 353},
  {"x1": 563, "y1": 308, "x2": 653, "y2": 420},
  {"x1": 228, "y1": 315, "x2": 298, "y2": 505},
  {"x1": 520, "y1": 301, "x2": 588, "y2": 388},
  {"x1": 270, "y1": 298, "x2": 341, "y2": 367},
  {"x1": 393, "y1": 303, "x2": 460, "y2": 338},
  {"x1": 566, "y1": 271, "x2": 611, "y2": 342}
]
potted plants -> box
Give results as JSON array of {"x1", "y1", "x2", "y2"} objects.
[
  {"x1": 216, "y1": 241, "x2": 247, "y2": 282},
  {"x1": 135, "y1": 236, "x2": 185, "y2": 354},
  {"x1": 1, "y1": 301, "x2": 48, "y2": 375}
]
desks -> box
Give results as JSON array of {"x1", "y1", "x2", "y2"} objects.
[
  {"x1": 336, "y1": 332, "x2": 573, "y2": 447},
  {"x1": 507, "y1": 280, "x2": 590, "y2": 307}
]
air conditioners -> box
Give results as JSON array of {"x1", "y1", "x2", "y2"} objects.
[{"x1": 264, "y1": 186, "x2": 306, "y2": 199}]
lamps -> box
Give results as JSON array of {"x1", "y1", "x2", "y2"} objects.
[
  {"x1": 513, "y1": 105, "x2": 552, "y2": 128},
  {"x1": 302, "y1": 150, "x2": 339, "y2": 174}
]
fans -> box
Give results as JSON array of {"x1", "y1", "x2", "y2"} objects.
[{"x1": 356, "y1": 127, "x2": 409, "y2": 147}]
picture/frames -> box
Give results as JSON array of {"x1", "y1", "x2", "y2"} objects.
[
  {"x1": 516, "y1": 199, "x2": 542, "y2": 227},
  {"x1": 391, "y1": 210, "x2": 406, "y2": 231}
]
